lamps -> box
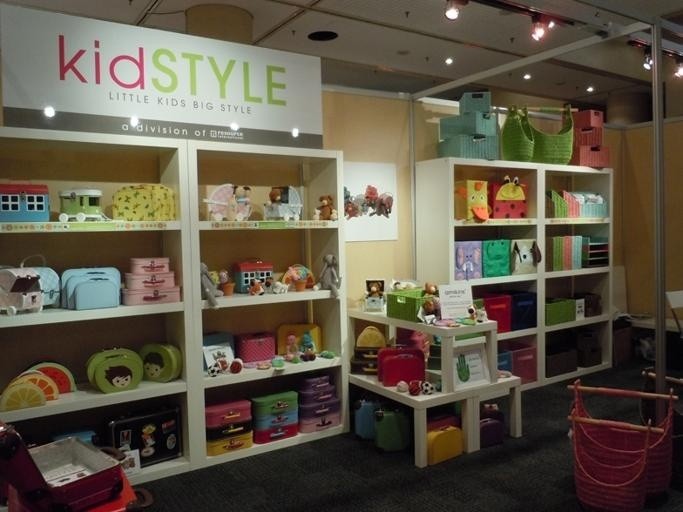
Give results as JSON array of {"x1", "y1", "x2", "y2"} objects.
[
  {"x1": 641, "y1": 44, "x2": 683, "y2": 81},
  {"x1": 440, "y1": 0, "x2": 548, "y2": 43}
]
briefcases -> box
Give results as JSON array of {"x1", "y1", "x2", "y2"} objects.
[
  {"x1": 0, "y1": 421, "x2": 156, "y2": 512},
  {"x1": 205, "y1": 368, "x2": 343, "y2": 458},
  {"x1": 85, "y1": 340, "x2": 183, "y2": 396},
  {"x1": 111, "y1": 402, "x2": 187, "y2": 469},
  {"x1": 378, "y1": 344, "x2": 428, "y2": 388},
  {"x1": 355, "y1": 395, "x2": 515, "y2": 468},
  {"x1": 1, "y1": 252, "x2": 185, "y2": 317}
]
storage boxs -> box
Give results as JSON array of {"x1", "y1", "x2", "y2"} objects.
[
  {"x1": 500, "y1": 289, "x2": 538, "y2": 331},
  {"x1": 561, "y1": 110, "x2": 611, "y2": 168},
  {"x1": 545, "y1": 187, "x2": 608, "y2": 218},
  {"x1": 453, "y1": 297, "x2": 487, "y2": 340},
  {"x1": 545, "y1": 346, "x2": 577, "y2": 378},
  {"x1": 497, "y1": 350, "x2": 512, "y2": 375},
  {"x1": 478, "y1": 291, "x2": 512, "y2": 334},
  {"x1": 385, "y1": 289, "x2": 440, "y2": 322},
  {"x1": 436, "y1": 90, "x2": 500, "y2": 161},
  {"x1": 545, "y1": 297, "x2": 577, "y2": 327},
  {"x1": 574, "y1": 292, "x2": 602, "y2": 317},
  {"x1": 572, "y1": 342, "x2": 603, "y2": 367},
  {"x1": 499, "y1": 341, "x2": 537, "y2": 384}
]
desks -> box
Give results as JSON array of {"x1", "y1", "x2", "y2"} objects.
[{"x1": 630, "y1": 315, "x2": 682, "y2": 336}]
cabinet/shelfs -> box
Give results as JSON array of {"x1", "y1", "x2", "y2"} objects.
[
  {"x1": 186, "y1": 138, "x2": 351, "y2": 469},
  {"x1": 0, "y1": 132, "x2": 198, "y2": 512},
  {"x1": 581, "y1": 235, "x2": 609, "y2": 267},
  {"x1": 344, "y1": 306, "x2": 523, "y2": 468},
  {"x1": 414, "y1": 155, "x2": 616, "y2": 396}
]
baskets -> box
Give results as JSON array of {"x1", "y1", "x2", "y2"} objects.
[
  {"x1": 565, "y1": 378, "x2": 679, "y2": 512},
  {"x1": 438, "y1": 90, "x2": 614, "y2": 170},
  {"x1": 545, "y1": 188, "x2": 608, "y2": 218}
]
